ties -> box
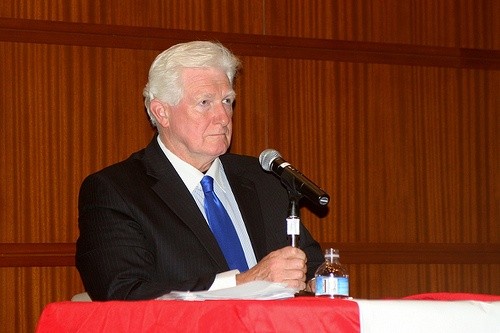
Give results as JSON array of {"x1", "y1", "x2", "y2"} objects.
[{"x1": 199, "y1": 175, "x2": 250, "y2": 274}]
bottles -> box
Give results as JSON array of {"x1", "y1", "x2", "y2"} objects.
[{"x1": 315, "y1": 249, "x2": 349, "y2": 299}]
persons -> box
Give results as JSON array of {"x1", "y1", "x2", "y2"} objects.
[{"x1": 75, "y1": 40, "x2": 327, "y2": 301}]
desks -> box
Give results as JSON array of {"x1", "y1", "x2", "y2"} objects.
[{"x1": 35, "y1": 293, "x2": 500, "y2": 333}]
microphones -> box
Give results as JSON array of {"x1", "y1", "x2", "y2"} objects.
[{"x1": 259, "y1": 149, "x2": 330, "y2": 207}]
